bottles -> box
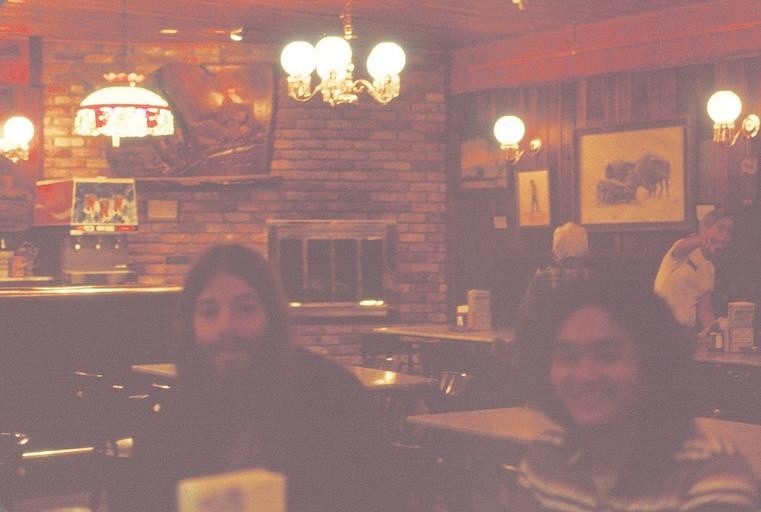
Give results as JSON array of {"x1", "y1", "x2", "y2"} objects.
[
  {"x1": 455, "y1": 305, "x2": 468, "y2": 329},
  {"x1": 707, "y1": 320, "x2": 725, "y2": 356}
]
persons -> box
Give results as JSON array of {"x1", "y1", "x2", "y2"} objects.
[
  {"x1": 86, "y1": 242, "x2": 410, "y2": 510},
  {"x1": 509, "y1": 269, "x2": 761, "y2": 512},
  {"x1": 652, "y1": 204, "x2": 737, "y2": 360},
  {"x1": 513, "y1": 219, "x2": 611, "y2": 347}
]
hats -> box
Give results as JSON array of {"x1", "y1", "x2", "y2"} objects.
[{"x1": 551, "y1": 220, "x2": 591, "y2": 262}]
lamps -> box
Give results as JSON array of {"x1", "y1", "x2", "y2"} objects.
[
  {"x1": 494, "y1": 113, "x2": 541, "y2": 167},
  {"x1": 282, "y1": 1, "x2": 407, "y2": 110},
  {"x1": 706, "y1": 91, "x2": 760, "y2": 174}
]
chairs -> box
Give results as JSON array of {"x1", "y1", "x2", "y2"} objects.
[{"x1": 77, "y1": 370, "x2": 150, "y2": 511}]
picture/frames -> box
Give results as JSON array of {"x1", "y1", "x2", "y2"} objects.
[
  {"x1": 451, "y1": 128, "x2": 551, "y2": 233},
  {"x1": 571, "y1": 114, "x2": 695, "y2": 236}
]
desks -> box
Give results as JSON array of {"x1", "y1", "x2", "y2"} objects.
[
  {"x1": 688, "y1": 342, "x2": 761, "y2": 381},
  {"x1": 133, "y1": 355, "x2": 437, "y2": 412},
  {"x1": 374, "y1": 324, "x2": 515, "y2": 373},
  {"x1": 401, "y1": 399, "x2": 761, "y2": 486}
]
